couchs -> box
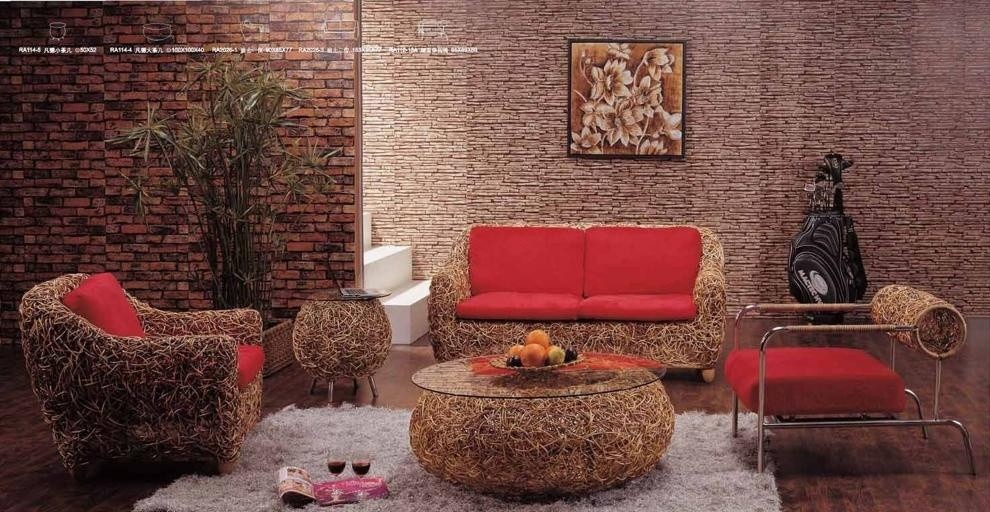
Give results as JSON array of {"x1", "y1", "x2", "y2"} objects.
[{"x1": 429, "y1": 221, "x2": 727, "y2": 383}]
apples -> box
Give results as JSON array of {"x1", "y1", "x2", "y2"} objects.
[{"x1": 547, "y1": 346, "x2": 566, "y2": 365}]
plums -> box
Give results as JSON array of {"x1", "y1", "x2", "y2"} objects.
[
  {"x1": 565, "y1": 345, "x2": 578, "y2": 362},
  {"x1": 507, "y1": 356, "x2": 520, "y2": 366}
]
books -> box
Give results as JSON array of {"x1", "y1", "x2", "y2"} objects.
[{"x1": 277, "y1": 466, "x2": 389, "y2": 506}]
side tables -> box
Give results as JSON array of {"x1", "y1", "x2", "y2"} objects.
[{"x1": 290, "y1": 288, "x2": 393, "y2": 403}]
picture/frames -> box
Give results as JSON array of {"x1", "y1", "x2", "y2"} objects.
[{"x1": 567, "y1": 36, "x2": 688, "y2": 158}]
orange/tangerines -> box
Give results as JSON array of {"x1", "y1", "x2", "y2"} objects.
[{"x1": 508, "y1": 345, "x2": 522, "y2": 358}]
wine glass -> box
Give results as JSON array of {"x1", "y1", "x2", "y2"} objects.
[{"x1": 326, "y1": 450, "x2": 372, "y2": 498}]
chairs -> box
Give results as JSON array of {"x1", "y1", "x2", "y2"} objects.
[
  {"x1": 723, "y1": 284, "x2": 977, "y2": 476},
  {"x1": 18, "y1": 271, "x2": 265, "y2": 482}
]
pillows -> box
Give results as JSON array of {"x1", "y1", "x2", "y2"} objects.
[
  {"x1": 468, "y1": 226, "x2": 702, "y2": 296},
  {"x1": 62, "y1": 272, "x2": 146, "y2": 338}
]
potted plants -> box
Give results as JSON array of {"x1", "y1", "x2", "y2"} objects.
[{"x1": 105, "y1": 42, "x2": 342, "y2": 378}]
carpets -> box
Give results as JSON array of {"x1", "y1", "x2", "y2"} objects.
[{"x1": 136, "y1": 403, "x2": 783, "y2": 512}]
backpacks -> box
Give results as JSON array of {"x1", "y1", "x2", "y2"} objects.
[{"x1": 787, "y1": 214, "x2": 868, "y2": 325}]
